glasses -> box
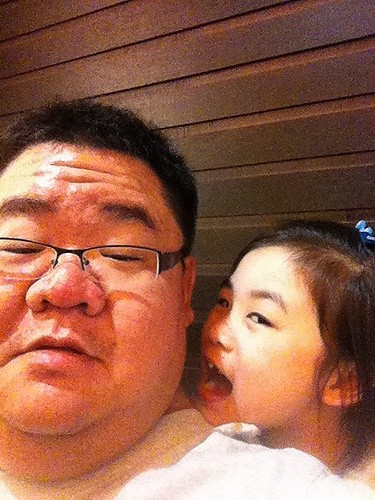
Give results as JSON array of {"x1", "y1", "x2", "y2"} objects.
[{"x1": 0, "y1": 236, "x2": 192, "y2": 283}]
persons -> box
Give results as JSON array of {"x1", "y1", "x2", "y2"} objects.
[
  {"x1": 196, "y1": 219, "x2": 375, "y2": 492},
  {"x1": 1, "y1": 98, "x2": 375, "y2": 500}
]
